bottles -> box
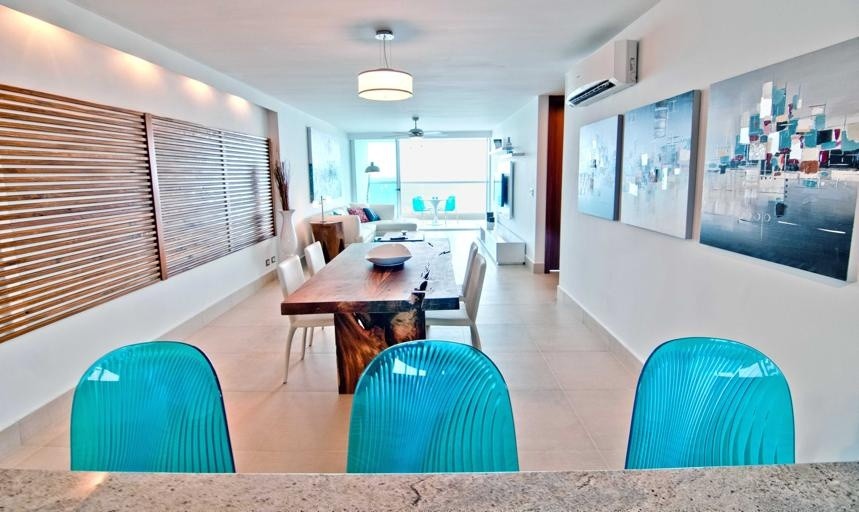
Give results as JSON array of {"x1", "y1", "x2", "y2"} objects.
[{"x1": 506, "y1": 137, "x2": 512, "y2": 153}]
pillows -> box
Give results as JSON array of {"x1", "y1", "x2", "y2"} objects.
[{"x1": 333, "y1": 205, "x2": 380, "y2": 224}]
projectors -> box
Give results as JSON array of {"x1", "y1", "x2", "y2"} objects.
[{"x1": 408, "y1": 129, "x2": 424, "y2": 137}]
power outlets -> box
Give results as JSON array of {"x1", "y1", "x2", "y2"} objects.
[
  {"x1": 271, "y1": 256, "x2": 275, "y2": 263},
  {"x1": 266, "y1": 259, "x2": 270, "y2": 267}
]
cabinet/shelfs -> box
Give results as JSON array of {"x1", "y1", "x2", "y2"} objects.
[
  {"x1": 476, "y1": 220, "x2": 525, "y2": 266},
  {"x1": 488, "y1": 147, "x2": 524, "y2": 162}
]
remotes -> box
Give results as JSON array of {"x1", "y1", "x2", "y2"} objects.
[{"x1": 391, "y1": 237, "x2": 408, "y2": 240}]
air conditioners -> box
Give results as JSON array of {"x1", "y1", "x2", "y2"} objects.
[{"x1": 565, "y1": 38, "x2": 638, "y2": 109}]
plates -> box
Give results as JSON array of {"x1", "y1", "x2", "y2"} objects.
[{"x1": 367, "y1": 243, "x2": 411, "y2": 266}]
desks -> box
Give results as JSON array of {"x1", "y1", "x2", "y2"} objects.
[
  {"x1": 309, "y1": 221, "x2": 345, "y2": 262},
  {"x1": 281, "y1": 238, "x2": 460, "y2": 394},
  {"x1": 418, "y1": 199, "x2": 449, "y2": 226},
  {"x1": 0, "y1": 461, "x2": 859, "y2": 512}
]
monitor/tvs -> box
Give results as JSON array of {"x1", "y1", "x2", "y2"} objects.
[{"x1": 494, "y1": 173, "x2": 508, "y2": 207}]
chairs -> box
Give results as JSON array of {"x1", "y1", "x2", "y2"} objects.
[
  {"x1": 304, "y1": 241, "x2": 335, "y2": 347},
  {"x1": 438, "y1": 194, "x2": 458, "y2": 224},
  {"x1": 276, "y1": 255, "x2": 336, "y2": 384},
  {"x1": 347, "y1": 340, "x2": 519, "y2": 472},
  {"x1": 626, "y1": 338, "x2": 796, "y2": 469},
  {"x1": 457, "y1": 240, "x2": 478, "y2": 302},
  {"x1": 424, "y1": 254, "x2": 486, "y2": 351},
  {"x1": 412, "y1": 196, "x2": 433, "y2": 225},
  {"x1": 70, "y1": 340, "x2": 235, "y2": 472}
]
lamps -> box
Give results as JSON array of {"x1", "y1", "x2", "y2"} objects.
[
  {"x1": 357, "y1": 29, "x2": 414, "y2": 101},
  {"x1": 311, "y1": 194, "x2": 332, "y2": 222},
  {"x1": 364, "y1": 162, "x2": 380, "y2": 205}
]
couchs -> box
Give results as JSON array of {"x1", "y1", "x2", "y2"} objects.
[{"x1": 310, "y1": 203, "x2": 418, "y2": 249}]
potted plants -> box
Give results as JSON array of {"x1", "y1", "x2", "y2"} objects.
[{"x1": 274, "y1": 159, "x2": 298, "y2": 280}]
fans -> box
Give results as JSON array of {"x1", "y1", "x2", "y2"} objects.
[{"x1": 383, "y1": 117, "x2": 440, "y2": 144}]
